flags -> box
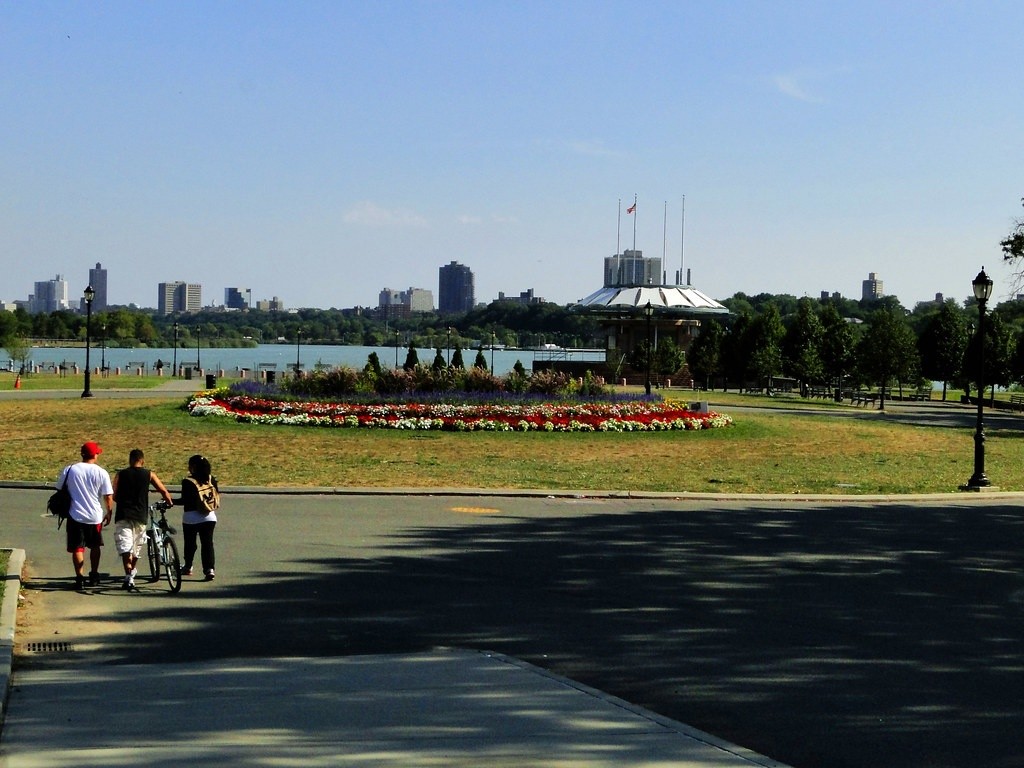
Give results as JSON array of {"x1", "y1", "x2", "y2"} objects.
[{"x1": 627, "y1": 203, "x2": 636, "y2": 214}]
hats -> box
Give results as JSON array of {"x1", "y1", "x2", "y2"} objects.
[{"x1": 80, "y1": 442, "x2": 103, "y2": 457}]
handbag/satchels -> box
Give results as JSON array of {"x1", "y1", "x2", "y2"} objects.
[{"x1": 47, "y1": 464, "x2": 72, "y2": 531}]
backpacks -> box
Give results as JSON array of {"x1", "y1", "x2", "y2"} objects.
[{"x1": 185, "y1": 474, "x2": 220, "y2": 512}]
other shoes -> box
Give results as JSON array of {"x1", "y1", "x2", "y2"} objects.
[
  {"x1": 122, "y1": 575, "x2": 135, "y2": 589},
  {"x1": 72, "y1": 577, "x2": 85, "y2": 592},
  {"x1": 130, "y1": 567, "x2": 137, "y2": 578},
  {"x1": 88, "y1": 572, "x2": 100, "y2": 585},
  {"x1": 205, "y1": 569, "x2": 215, "y2": 580},
  {"x1": 181, "y1": 565, "x2": 193, "y2": 575}
]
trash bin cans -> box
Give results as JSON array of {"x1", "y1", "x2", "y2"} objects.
[
  {"x1": 835, "y1": 389, "x2": 844, "y2": 403},
  {"x1": 206, "y1": 374, "x2": 216, "y2": 390},
  {"x1": 267, "y1": 371, "x2": 276, "y2": 383},
  {"x1": 185, "y1": 367, "x2": 192, "y2": 380}
]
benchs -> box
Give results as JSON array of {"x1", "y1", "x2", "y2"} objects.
[
  {"x1": 153, "y1": 362, "x2": 170, "y2": 369},
  {"x1": 811, "y1": 385, "x2": 833, "y2": 400},
  {"x1": 872, "y1": 388, "x2": 893, "y2": 400},
  {"x1": 38, "y1": 361, "x2": 54, "y2": 370},
  {"x1": 259, "y1": 363, "x2": 277, "y2": 371},
  {"x1": 909, "y1": 387, "x2": 933, "y2": 402},
  {"x1": 60, "y1": 361, "x2": 76, "y2": 368},
  {"x1": 127, "y1": 361, "x2": 145, "y2": 369},
  {"x1": 1005, "y1": 395, "x2": 1024, "y2": 412},
  {"x1": 286, "y1": 362, "x2": 304, "y2": 371},
  {"x1": 851, "y1": 390, "x2": 873, "y2": 407},
  {"x1": 179, "y1": 361, "x2": 198, "y2": 369}
]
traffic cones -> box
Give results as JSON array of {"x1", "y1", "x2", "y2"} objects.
[{"x1": 14, "y1": 374, "x2": 22, "y2": 390}]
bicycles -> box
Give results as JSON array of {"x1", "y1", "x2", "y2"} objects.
[{"x1": 143, "y1": 499, "x2": 182, "y2": 594}]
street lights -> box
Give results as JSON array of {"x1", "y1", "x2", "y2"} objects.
[
  {"x1": 296, "y1": 327, "x2": 301, "y2": 369},
  {"x1": 970, "y1": 265, "x2": 994, "y2": 487},
  {"x1": 196, "y1": 324, "x2": 201, "y2": 369},
  {"x1": 446, "y1": 326, "x2": 452, "y2": 366},
  {"x1": 81, "y1": 285, "x2": 95, "y2": 398},
  {"x1": 172, "y1": 322, "x2": 179, "y2": 377},
  {"x1": 491, "y1": 331, "x2": 496, "y2": 378},
  {"x1": 395, "y1": 329, "x2": 399, "y2": 367},
  {"x1": 644, "y1": 302, "x2": 654, "y2": 396}
]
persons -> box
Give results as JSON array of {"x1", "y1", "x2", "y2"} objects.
[
  {"x1": 161, "y1": 455, "x2": 218, "y2": 581},
  {"x1": 112, "y1": 449, "x2": 172, "y2": 590},
  {"x1": 56, "y1": 442, "x2": 112, "y2": 593},
  {"x1": 157, "y1": 359, "x2": 163, "y2": 369}
]
